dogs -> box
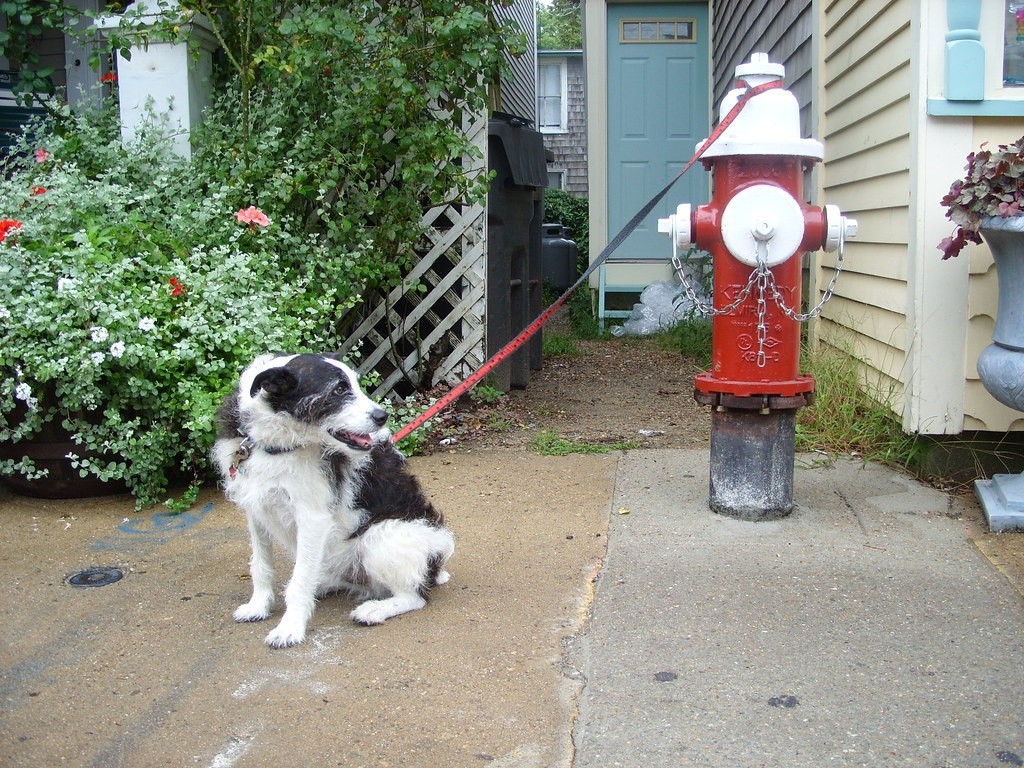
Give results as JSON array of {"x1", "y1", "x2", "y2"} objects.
[{"x1": 209, "y1": 352, "x2": 456, "y2": 651}]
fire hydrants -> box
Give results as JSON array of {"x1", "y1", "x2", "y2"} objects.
[{"x1": 657, "y1": 53, "x2": 858, "y2": 524}]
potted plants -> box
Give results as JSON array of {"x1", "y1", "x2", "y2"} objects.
[{"x1": 937, "y1": 135, "x2": 1024, "y2": 532}]
